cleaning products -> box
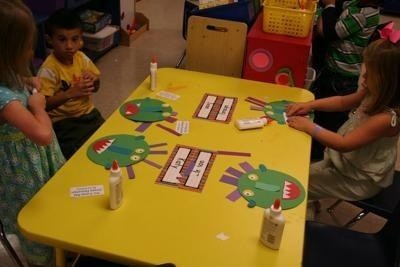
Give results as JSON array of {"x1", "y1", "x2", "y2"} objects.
[
  {"x1": 109, "y1": 158, "x2": 124, "y2": 209},
  {"x1": 236, "y1": 117, "x2": 273, "y2": 130},
  {"x1": 260, "y1": 198, "x2": 285, "y2": 251},
  {"x1": 149, "y1": 55, "x2": 158, "y2": 91}
]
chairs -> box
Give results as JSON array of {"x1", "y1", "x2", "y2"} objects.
[
  {"x1": 174, "y1": 16, "x2": 248, "y2": 80},
  {"x1": 306, "y1": 170, "x2": 400, "y2": 267}
]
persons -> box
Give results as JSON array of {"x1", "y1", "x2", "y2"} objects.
[
  {"x1": 317, "y1": 0, "x2": 380, "y2": 94},
  {"x1": 0, "y1": 0, "x2": 106, "y2": 267},
  {"x1": 286, "y1": 38, "x2": 400, "y2": 201}
]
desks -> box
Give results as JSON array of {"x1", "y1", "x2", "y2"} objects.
[{"x1": 15, "y1": 66, "x2": 318, "y2": 266}]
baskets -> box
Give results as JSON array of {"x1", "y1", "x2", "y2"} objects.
[{"x1": 261, "y1": 0, "x2": 318, "y2": 38}]
